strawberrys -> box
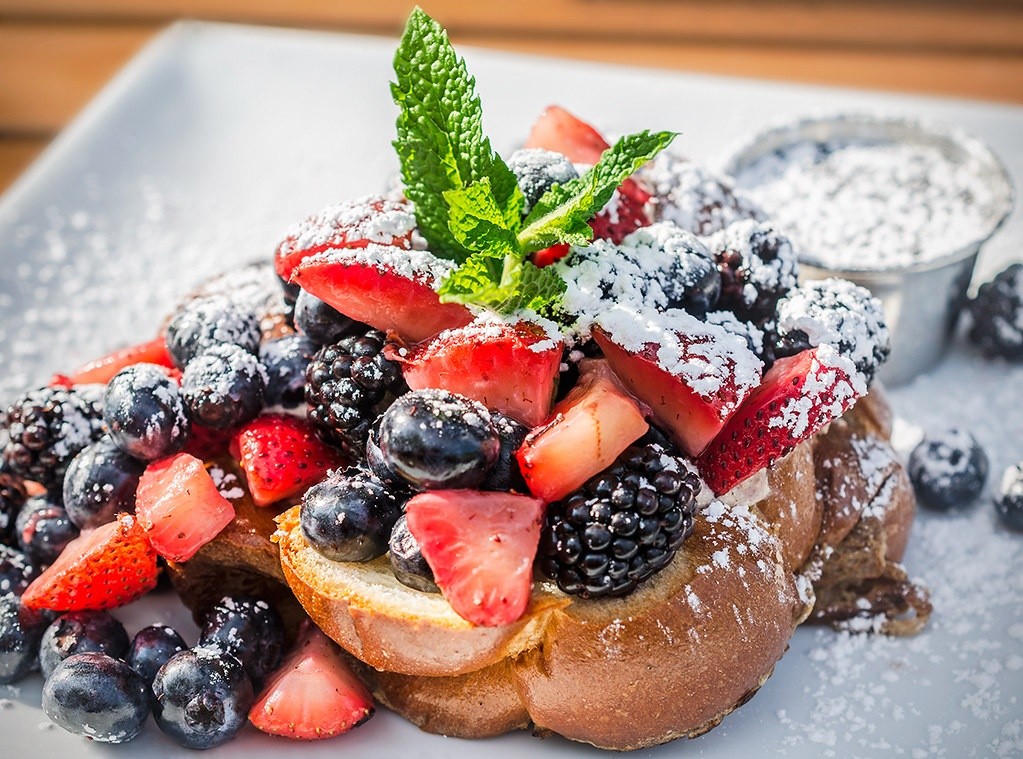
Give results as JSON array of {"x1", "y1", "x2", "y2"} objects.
[{"x1": 21, "y1": 107, "x2": 861, "y2": 742}]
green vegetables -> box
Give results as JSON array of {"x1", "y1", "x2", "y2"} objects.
[{"x1": 388, "y1": 3, "x2": 680, "y2": 314}]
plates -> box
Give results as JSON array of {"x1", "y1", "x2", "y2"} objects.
[{"x1": 0, "y1": 18, "x2": 1023, "y2": 758}]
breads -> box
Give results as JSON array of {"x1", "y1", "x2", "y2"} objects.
[{"x1": 176, "y1": 314, "x2": 913, "y2": 753}]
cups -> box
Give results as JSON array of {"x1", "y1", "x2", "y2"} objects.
[{"x1": 724, "y1": 106, "x2": 1016, "y2": 386}]
